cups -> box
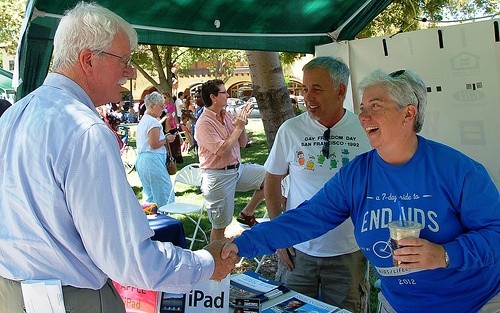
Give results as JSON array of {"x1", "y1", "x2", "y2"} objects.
[{"x1": 389, "y1": 219, "x2": 424, "y2": 269}]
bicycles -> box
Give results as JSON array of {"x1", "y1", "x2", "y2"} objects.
[{"x1": 118, "y1": 125, "x2": 137, "y2": 176}]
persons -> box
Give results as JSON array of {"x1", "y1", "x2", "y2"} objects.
[
  {"x1": 112, "y1": 106, "x2": 138, "y2": 122},
  {"x1": 136, "y1": 92, "x2": 175, "y2": 208},
  {"x1": 162, "y1": 89, "x2": 207, "y2": 163},
  {"x1": 0, "y1": 2, "x2": 241, "y2": 313},
  {"x1": 221, "y1": 68, "x2": 500, "y2": 313},
  {"x1": 194, "y1": 79, "x2": 264, "y2": 244},
  {"x1": 264, "y1": 56, "x2": 375, "y2": 313},
  {"x1": 290, "y1": 91, "x2": 298, "y2": 103}
]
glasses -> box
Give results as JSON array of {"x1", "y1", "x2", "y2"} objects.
[
  {"x1": 388, "y1": 69, "x2": 420, "y2": 114},
  {"x1": 322, "y1": 127, "x2": 330, "y2": 159},
  {"x1": 218, "y1": 90, "x2": 227, "y2": 94},
  {"x1": 92, "y1": 49, "x2": 133, "y2": 66}
]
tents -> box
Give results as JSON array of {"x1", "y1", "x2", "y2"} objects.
[{"x1": 12, "y1": 0, "x2": 396, "y2": 103}]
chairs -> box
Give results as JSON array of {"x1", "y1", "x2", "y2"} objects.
[
  {"x1": 178, "y1": 125, "x2": 195, "y2": 154},
  {"x1": 158, "y1": 162, "x2": 208, "y2": 250}
]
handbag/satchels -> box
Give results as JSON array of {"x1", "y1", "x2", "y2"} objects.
[{"x1": 165, "y1": 137, "x2": 177, "y2": 175}]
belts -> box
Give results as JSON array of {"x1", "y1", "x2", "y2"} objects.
[{"x1": 220, "y1": 163, "x2": 240, "y2": 170}]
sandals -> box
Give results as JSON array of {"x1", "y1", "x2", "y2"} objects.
[{"x1": 236, "y1": 212, "x2": 259, "y2": 228}]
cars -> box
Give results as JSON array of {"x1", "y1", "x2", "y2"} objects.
[{"x1": 222, "y1": 94, "x2": 306, "y2": 118}]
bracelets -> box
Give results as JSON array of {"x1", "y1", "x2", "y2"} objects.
[{"x1": 445, "y1": 252, "x2": 449, "y2": 266}]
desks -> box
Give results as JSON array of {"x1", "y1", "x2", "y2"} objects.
[{"x1": 145, "y1": 211, "x2": 186, "y2": 249}]
